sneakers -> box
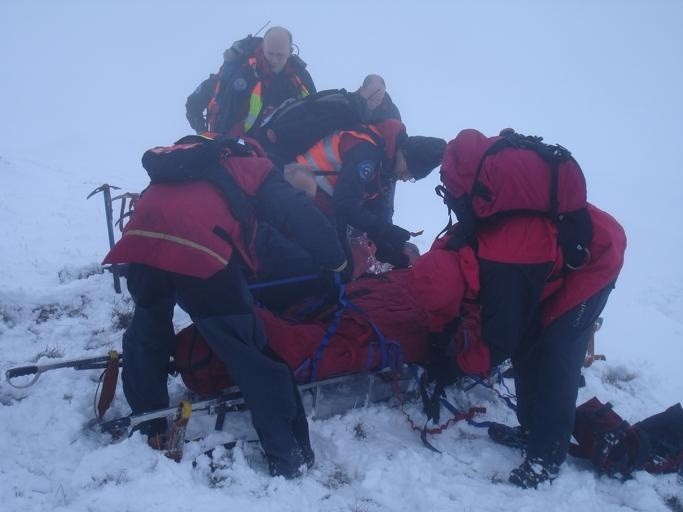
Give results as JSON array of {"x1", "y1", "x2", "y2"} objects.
[
  {"x1": 487, "y1": 416, "x2": 530, "y2": 449},
  {"x1": 508, "y1": 460, "x2": 559, "y2": 491}
]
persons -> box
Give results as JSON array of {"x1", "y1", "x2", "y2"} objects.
[
  {"x1": 185, "y1": 24, "x2": 317, "y2": 142},
  {"x1": 355, "y1": 74, "x2": 400, "y2": 127},
  {"x1": 256, "y1": 117, "x2": 449, "y2": 310},
  {"x1": 398, "y1": 203, "x2": 628, "y2": 491},
  {"x1": 120, "y1": 131, "x2": 350, "y2": 477}
]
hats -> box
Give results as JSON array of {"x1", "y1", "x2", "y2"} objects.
[{"x1": 402, "y1": 135, "x2": 446, "y2": 182}]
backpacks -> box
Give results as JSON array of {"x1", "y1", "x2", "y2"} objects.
[
  {"x1": 437, "y1": 124, "x2": 589, "y2": 244},
  {"x1": 137, "y1": 129, "x2": 258, "y2": 220},
  {"x1": 255, "y1": 86, "x2": 390, "y2": 167},
  {"x1": 219, "y1": 33, "x2": 264, "y2": 89}
]
